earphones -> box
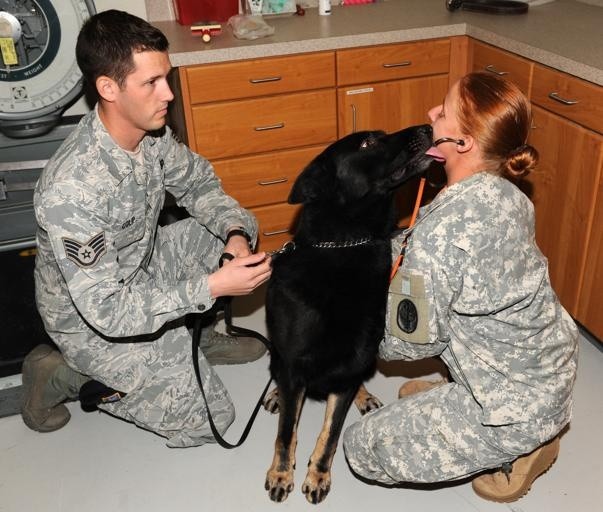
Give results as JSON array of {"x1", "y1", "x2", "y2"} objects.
[{"x1": 459, "y1": 139, "x2": 464, "y2": 145}]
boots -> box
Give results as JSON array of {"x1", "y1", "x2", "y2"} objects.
[
  {"x1": 19, "y1": 345, "x2": 93, "y2": 433},
  {"x1": 187, "y1": 315, "x2": 267, "y2": 368}
]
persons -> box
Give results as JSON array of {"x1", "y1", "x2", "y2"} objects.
[
  {"x1": 341, "y1": 69, "x2": 580, "y2": 503},
  {"x1": 17, "y1": 8, "x2": 273, "y2": 452}
]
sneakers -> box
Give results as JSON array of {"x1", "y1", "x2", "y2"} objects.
[
  {"x1": 398, "y1": 377, "x2": 450, "y2": 399},
  {"x1": 472, "y1": 435, "x2": 560, "y2": 503}
]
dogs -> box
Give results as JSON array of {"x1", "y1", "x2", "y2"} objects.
[{"x1": 257, "y1": 122, "x2": 447, "y2": 506}]
